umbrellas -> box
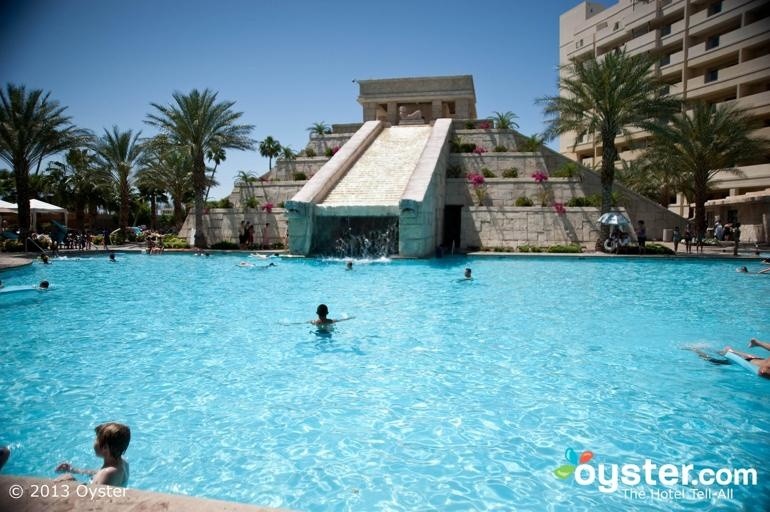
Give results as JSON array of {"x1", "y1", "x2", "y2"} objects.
[{"x1": 597, "y1": 211, "x2": 630, "y2": 226}]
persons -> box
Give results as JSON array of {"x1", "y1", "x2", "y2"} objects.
[
  {"x1": 682, "y1": 338, "x2": 769, "y2": 379},
  {"x1": 238, "y1": 218, "x2": 289, "y2": 248},
  {"x1": 636, "y1": 219, "x2": 649, "y2": 250},
  {"x1": 0, "y1": 217, "x2": 165, "y2": 264},
  {"x1": 672, "y1": 222, "x2": 770, "y2": 277},
  {"x1": 603, "y1": 229, "x2": 631, "y2": 255},
  {"x1": 32, "y1": 280, "x2": 49, "y2": 291},
  {"x1": 346, "y1": 260, "x2": 354, "y2": 270},
  {"x1": 289, "y1": 303, "x2": 356, "y2": 326},
  {"x1": 53, "y1": 422, "x2": 131, "y2": 487},
  {"x1": 463, "y1": 267, "x2": 472, "y2": 280}
]
